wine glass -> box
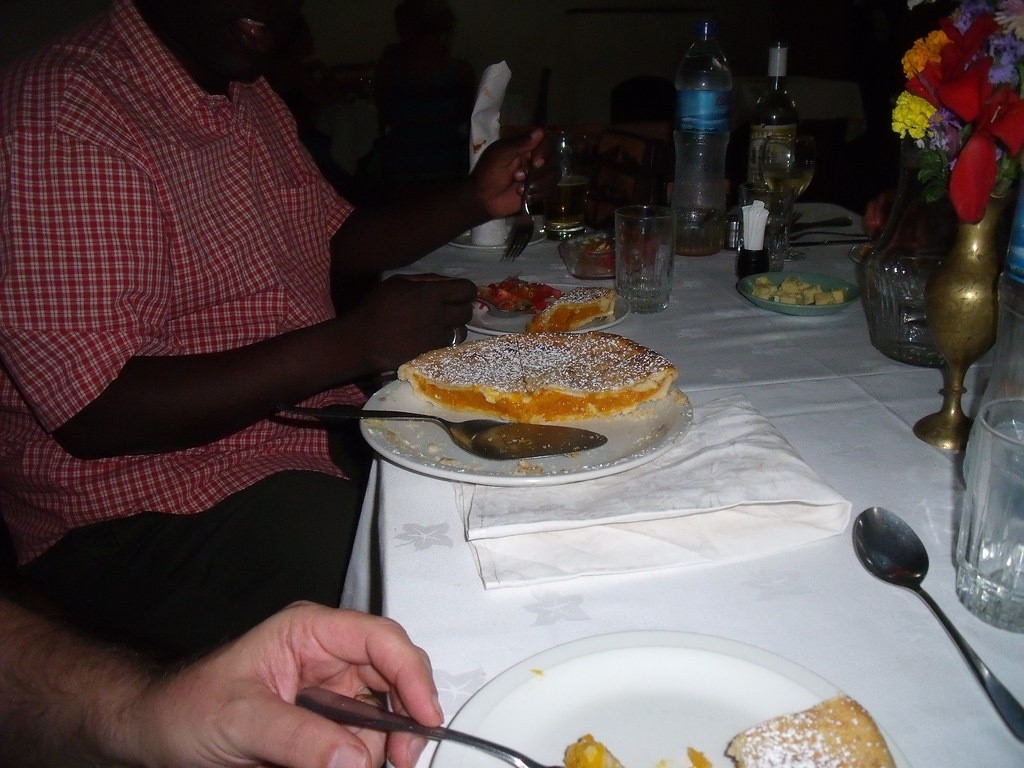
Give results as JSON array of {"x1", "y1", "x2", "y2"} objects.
[{"x1": 763, "y1": 134, "x2": 816, "y2": 261}]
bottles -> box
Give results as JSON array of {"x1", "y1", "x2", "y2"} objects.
[
  {"x1": 670, "y1": 19, "x2": 734, "y2": 257},
  {"x1": 962, "y1": 184, "x2": 1023, "y2": 492},
  {"x1": 727, "y1": 216, "x2": 740, "y2": 250},
  {"x1": 746, "y1": 40, "x2": 797, "y2": 183}
]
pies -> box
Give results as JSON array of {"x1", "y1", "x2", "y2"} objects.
[
  {"x1": 396, "y1": 330, "x2": 678, "y2": 424},
  {"x1": 527, "y1": 285, "x2": 617, "y2": 333},
  {"x1": 726, "y1": 695, "x2": 897, "y2": 768}
]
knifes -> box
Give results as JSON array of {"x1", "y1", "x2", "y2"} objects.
[{"x1": 789, "y1": 239, "x2": 869, "y2": 247}]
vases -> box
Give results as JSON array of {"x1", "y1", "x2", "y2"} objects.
[{"x1": 914, "y1": 190, "x2": 1012, "y2": 449}]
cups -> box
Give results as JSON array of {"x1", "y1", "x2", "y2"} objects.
[
  {"x1": 734, "y1": 191, "x2": 792, "y2": 271},
  {"x1": 473, "y1": 216, "x2": 505, "y2": 245},
  {"x1": 955, "y1": 399, "x2": 1024, "y2": 634},
  {"x1": 541, "y1": 130, "x2": 596, "y2": 240},
  {"x1": 614, "y1": 205, "x2": 681, "y2": 314}
]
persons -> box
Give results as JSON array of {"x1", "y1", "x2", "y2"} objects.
[
  {"x1": 284, "y1": 23, "x2": 327, "y2": 110},
  {"x1": 379, "y1": 2, "x2": 474, "y2": 173},
  {"x1": 0, "y1": 0, "x2": 562, "y2": 768}
]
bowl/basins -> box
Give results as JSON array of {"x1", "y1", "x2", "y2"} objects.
[{"x1": 557, "y1": 229, "x2": 615, "y2": 278}]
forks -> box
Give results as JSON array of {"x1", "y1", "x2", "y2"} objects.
[
  {"x1": 504, "y1": 159, "x2": 534, "y2": 260},
  {"x1": 792, "y1": 211, "x2": 803, "y2": 223}
]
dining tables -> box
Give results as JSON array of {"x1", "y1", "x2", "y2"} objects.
[{"x1": 341, "y1": 203, "x2": 1024, "y2": 768}]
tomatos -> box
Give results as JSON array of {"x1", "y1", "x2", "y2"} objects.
[{"x1": 478, "y1": 275, "x2": 562, "y2": 313}]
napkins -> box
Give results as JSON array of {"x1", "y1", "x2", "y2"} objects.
[
  {"x1": 454, "y1": 393, "x2": 852, "y2": 589},
  {"x1": 469, "y1": 59, "x2": 512, "y2": 245}
]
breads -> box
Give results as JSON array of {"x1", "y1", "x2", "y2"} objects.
[{"x1": 751, "y1": 275, "x2": 845, "y2": 305}]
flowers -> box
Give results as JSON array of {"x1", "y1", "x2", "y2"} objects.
[{"x1": 891, "y1": 0, "x2": 1024, "y2": 227}]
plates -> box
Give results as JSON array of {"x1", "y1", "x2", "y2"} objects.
[
  {"x1": 463, "y1": 284, "x2": 631, "y2": 334},
  {"x1": 359, "y1": 378, "x2": 693, "y2": 487},
  {"x1": 447, "y1": 223, "x2": 546, "y2": 251},
  {"x1": 428, "y1": 630, "x2": 913, "y2": 767},
  {"x1": 736, "y1": 270, "x2": 862, "y2": 315}
]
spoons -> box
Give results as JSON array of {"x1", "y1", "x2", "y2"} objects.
[
  {"x1": 853, "y1": 507, "x2": 1024, "y2": 742},
  {"x1": 476, "y1": 297, "x2": 528, "y2": 318},
  {"x1": 275, "y1": 403, "x2": 609, "y2": 460}
]
